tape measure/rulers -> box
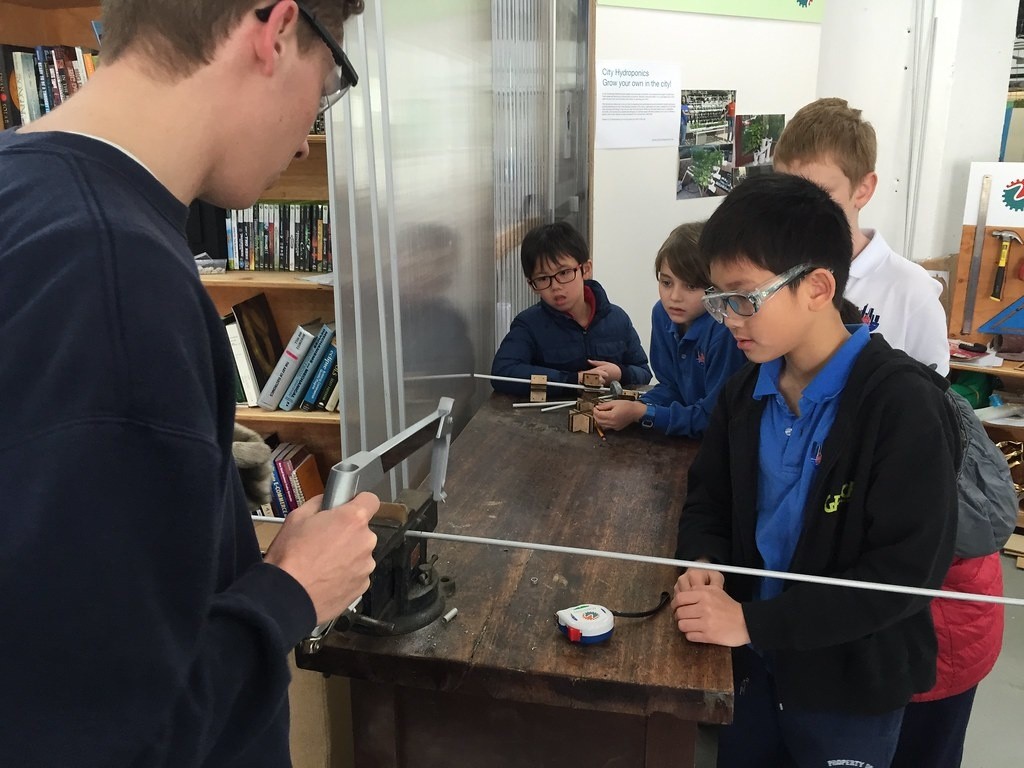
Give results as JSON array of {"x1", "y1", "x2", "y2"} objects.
[
  {"x1": 553, "y1": 602, "x2": 616, "y2": 645},
  {"x1": 959, "y1": 174, "x2": 993, "y2": 336}
]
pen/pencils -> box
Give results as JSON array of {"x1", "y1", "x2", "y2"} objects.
[{"x1": 593, "y1": 420, "x2": 607, "y2": 441}]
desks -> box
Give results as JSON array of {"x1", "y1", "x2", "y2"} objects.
[{"x1": 294, "y1": 384, "x2": 734, "y2": 768}]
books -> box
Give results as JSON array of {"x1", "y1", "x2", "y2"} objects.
[
  {"x1": 0, "y1": 20, "x2": 101, "y2": 131},
  {"x1": 186, "y1": 198, "x2": 332, "y2": 273},
  {"x1": 221, "y1": 293, "x2": 340, "y2": 416},
  {"x1": 245, "y1": 430, "x2": 324, "y2": 520}
]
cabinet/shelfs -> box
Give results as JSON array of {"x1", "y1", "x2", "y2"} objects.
[
  {"x1": 0, "y1": 0, "x2": 343, "y2": 552},
  {"x1": 938, "y1": 353, "x2": 1024, "y2": 557}
]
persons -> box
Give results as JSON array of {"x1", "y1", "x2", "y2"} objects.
[
  {"x1": 1, "y1": 0, "x2": 381, "y2": 768},
  {"x1": 670, "y1": 172, "x2": 962, "y2": 768},
  {"x1": 774, "y1": 99, "x2": 951, "y2": 382},
  {"x1": 593, "y1": 222, "x2": 743, "y2": 441},
  {"x1": 838, "y1": 298, "x2": 1017, "y2": 767},
  {"x1": 489, "y1": 221, "x2": 651, "y2": 397}
]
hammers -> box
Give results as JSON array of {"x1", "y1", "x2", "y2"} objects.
[{"x1": 988, "y1": 228, "x2": 1024, "y2": 303}]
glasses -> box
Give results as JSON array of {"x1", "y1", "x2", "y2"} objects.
[
  {"x1": 255, "y1": 1, "x2": 359, "y2": 116},
  {"x1": 528, "y1": 263, "x2": 584, "y2": 291},
  {"x1": 701, "y1": 263, "x2": 834, "y2": 325}
]
